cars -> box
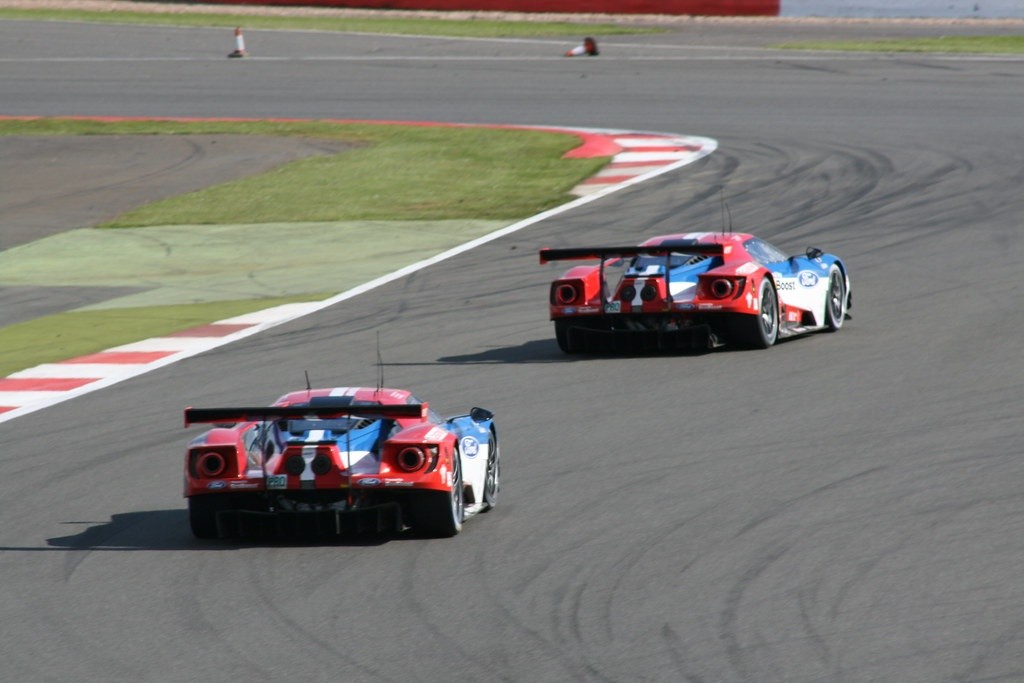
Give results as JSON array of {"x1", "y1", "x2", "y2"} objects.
[
  {"x1": 183, "y1": 388, "x2": 499, "y2": 548},
  {"x1": 540, "y1": 231, "x2": 852, "y2": 353}
]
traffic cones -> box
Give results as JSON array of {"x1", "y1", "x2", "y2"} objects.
[
  {"x1": 566, "y1": 37, "x2": 598, "y2": 56},
  {"x1": 227, "y1": 28, "x2": 248, "y2": 57}
]
